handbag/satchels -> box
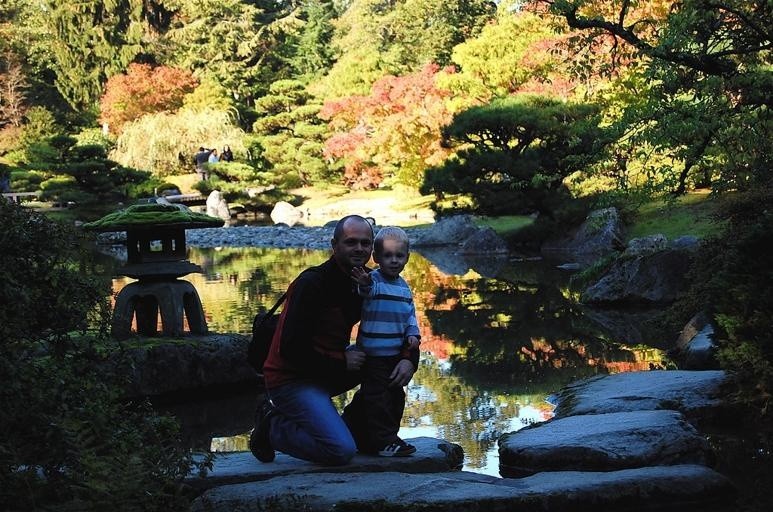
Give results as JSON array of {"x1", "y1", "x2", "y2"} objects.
[{"x1": 247, "y1": 291, "x2": 287, "y2": 379}]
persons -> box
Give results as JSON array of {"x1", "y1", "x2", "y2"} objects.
[
  {"x1": 207, "y1": 148, "x2": 219, "y2": 164},
  {"x1": 347, "y1": 224, "x2": 422, "y2": 460},
  {"x1": 191, "y1": 146, "x2": 212, "y2": 182},
  {"x1": 246, "y1": 215, "x2": 423, "y2": 465},
  {"x1": 219, "y1": 144, "x2": 233, "y2": 184}
]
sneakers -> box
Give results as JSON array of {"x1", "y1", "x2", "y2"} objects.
[
  {"x1": 249, "y1": 400, "x2": 275, "y2": 463},
  {"x1": 378, "y1": 443, "x2": 417, "y2": 457}
]
